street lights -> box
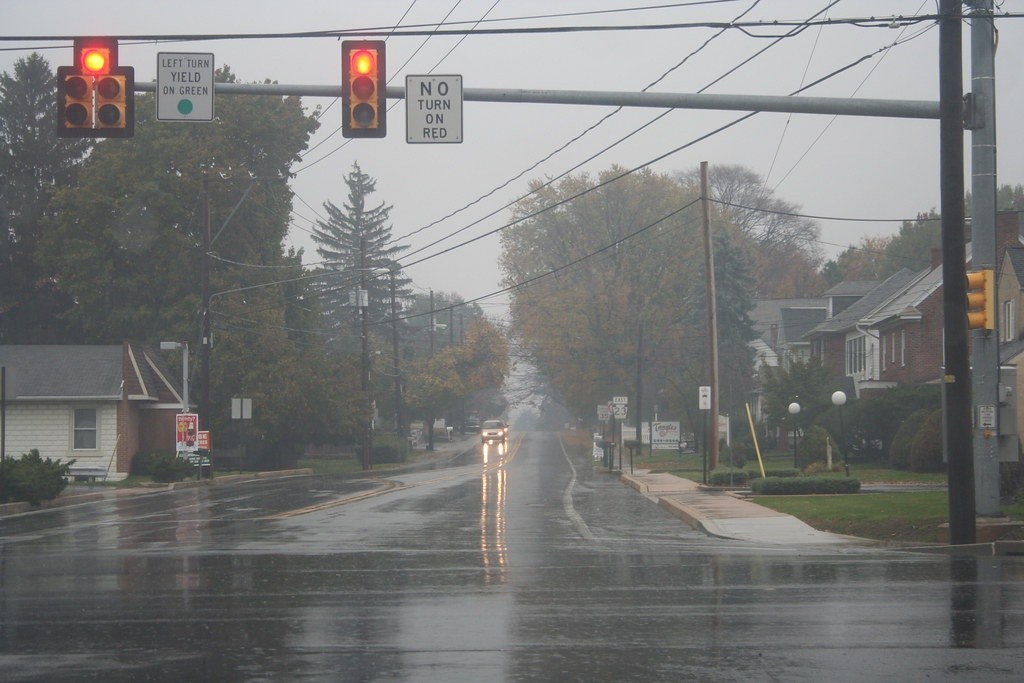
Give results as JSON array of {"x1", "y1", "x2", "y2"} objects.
[
  {"x1": 831, "y1": 390, "x2": 851, "y2": 476},
  {"x1": 159, "y1": 341, "x2": 189, "y2": 412},
  {"x1": 788, "y1": 403, "x2": 801, "y2": 467}
]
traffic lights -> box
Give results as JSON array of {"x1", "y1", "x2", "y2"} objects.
[
  {"x1": 56, "y1": 36, "x2": 135, "y2": 139},
  {"x1": 965, "y1": 268, "x2": 995, "y2": 334},
  {"x1": 341, "y1": 39, "x2": 386, "y2": 139}
]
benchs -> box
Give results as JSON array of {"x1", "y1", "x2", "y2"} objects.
[{"x1": 68, "y1": 467, "x2": 107, "y2": 483}]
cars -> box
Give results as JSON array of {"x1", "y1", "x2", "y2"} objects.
[
  {"x1": 480, "y1": 420, "x2": 509, "y2": 443},
  {"x1": 465, "y1": 421, "x2": 481, "y2": 433}
]
